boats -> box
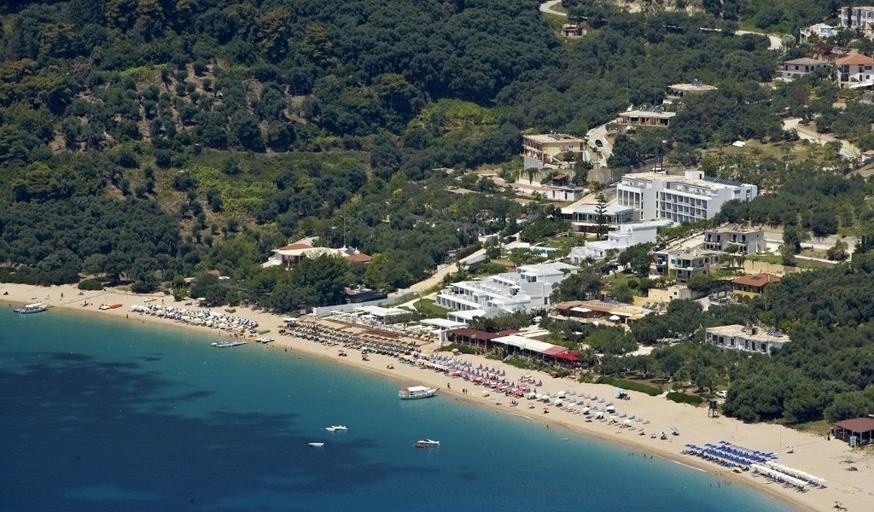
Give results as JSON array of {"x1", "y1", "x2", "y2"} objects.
[
  {"x1": 398, "y1": 386, "x2": 437, "y2": 399},
  {"x1": 308, "y1": 442, "x2": 324, "y2": 447},
  {"x1": 209, "y1": 337, "x2": 273, "y2": 348},
  {"x1": 13, "y1": 303, "x2": 46, "y2": 314},
  {"x1": 332, "y1": 425, "x2": 347, "y2": 429},
  {"x1": 325, "y1": 427, "x2": 335, "y2": 431},
  {"x1": 415, "y1": 439, "x2": 439, "y2": 448}
]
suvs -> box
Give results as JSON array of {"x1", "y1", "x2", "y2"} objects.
[{"x1": 716, "y1": 390, "x2": 727, "y2": 399}]
